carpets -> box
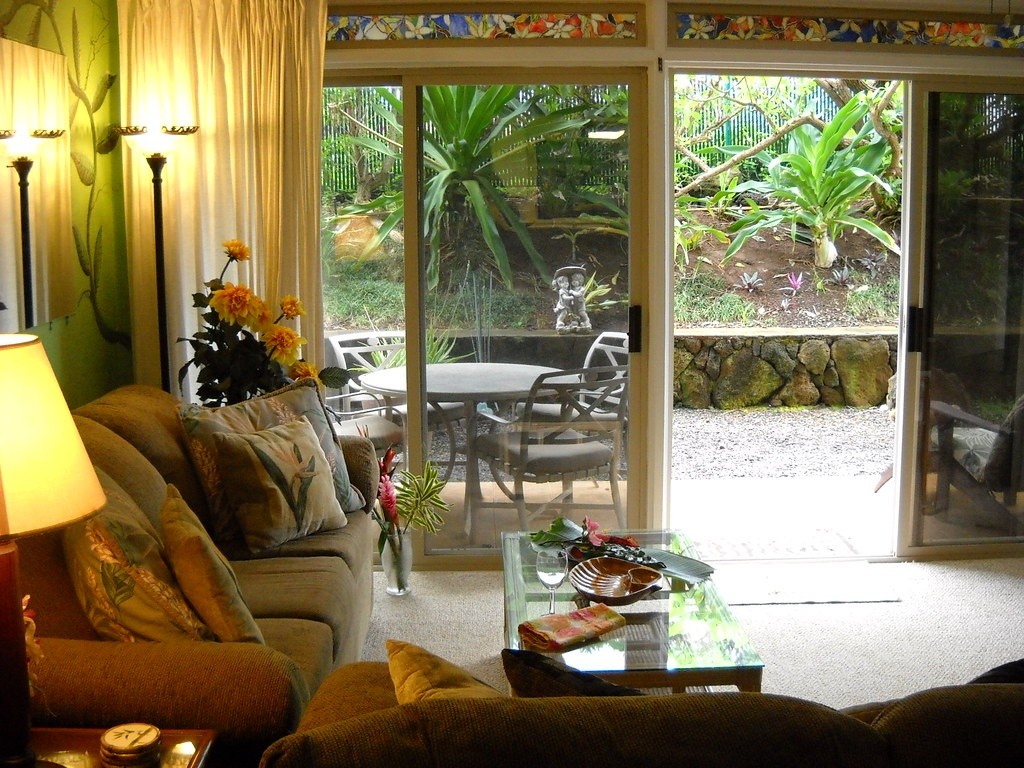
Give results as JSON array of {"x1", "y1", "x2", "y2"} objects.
[{"x1": 708, "y1": 559, "x2": 903, "y2": 605}]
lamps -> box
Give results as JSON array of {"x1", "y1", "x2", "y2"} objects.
[
  {"x1": 109, "y1": 122, "x2": 200, "y2": 392},
  {"x1": 0, "y1": 128, "x2": 66, "y2": 329},
  {"x1": 0, "y1": 333, "x2": 109, "y2": 768}
]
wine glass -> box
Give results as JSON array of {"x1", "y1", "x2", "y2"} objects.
[{"x1": 536, "y1": 549, "x2": 569, "y2": 619}]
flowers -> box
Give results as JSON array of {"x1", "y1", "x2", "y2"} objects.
[
  {"x1": 171, "y1": 238, "x2": 352, "y2": 412},
  {"x1": 365, "y1": 441, "x2": 452, "y2": 591},
  {"x1": 537, "y1": 518, "x2": 603, "y2": 548}
]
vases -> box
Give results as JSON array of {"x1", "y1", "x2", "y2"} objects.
[{"x1": 380, "y1": 527, "x2": 412, "y2": 597}]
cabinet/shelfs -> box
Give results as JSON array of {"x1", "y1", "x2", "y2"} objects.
[{"x1": 27, "y1": 726, "x2": 219, "y2": 768}]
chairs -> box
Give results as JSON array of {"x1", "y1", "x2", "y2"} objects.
[
  {"x1": 470, "y1": 364, "x2": 629, "y2": 539},
  {"x1": 323, "y1": 394, "x2": 407, "y2": 477},
  {"x1": 329, "y1": 328, "x2": 474, "y2": 448},
  {"x1": 923, "y1": 401, "x2": 1022, "y2": 529},
  {"x1": 516, "y1": 326, "x2": 631, "y2": 484}
]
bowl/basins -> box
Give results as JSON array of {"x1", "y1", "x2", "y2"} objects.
[{"x1": 568, "y1": 556, "x2": 663, "y2": 605}]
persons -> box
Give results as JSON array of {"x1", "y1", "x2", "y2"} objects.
[{"x1": 552, "y1": 273, "x2": 591, "y2": 327}]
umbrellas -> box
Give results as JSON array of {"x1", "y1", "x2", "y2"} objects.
[{"x1": 554, "y1": 266, "x2": 587, "y2": 286}]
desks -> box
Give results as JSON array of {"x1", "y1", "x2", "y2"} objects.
[
  {"x1": 499, "y1": 527, "x2": 764, "y2": 695},
  {"x1": 358, "y1": 362, "x2": 584, "y2": 513}
]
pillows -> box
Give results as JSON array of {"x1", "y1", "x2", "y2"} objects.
[
  {"x1": 63, "y1": 460, "x2": 215, "y2": 646},
  {"x1": 175, "y1": 377, "x2": 369, "y2": 553},
  {"x1": 162, "y1": 481, "x2": 267, "y2": 651},
  {"x1": 499, "y1": 646, "x2": 649, "y2": 697},
  {"x1": 382, "y1": 638, "x2": 506, "y2": 708},
  {"x1": 211, "y1": 413, "x2": 349, "y2": 555}
]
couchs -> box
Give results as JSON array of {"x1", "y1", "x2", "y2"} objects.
[
  {"x1": 258, "y1": 658, "x2": 1024, "y2": 768},
  {"x1": 15, "y1": 381, "x2": 380, "y2": 766}
]
